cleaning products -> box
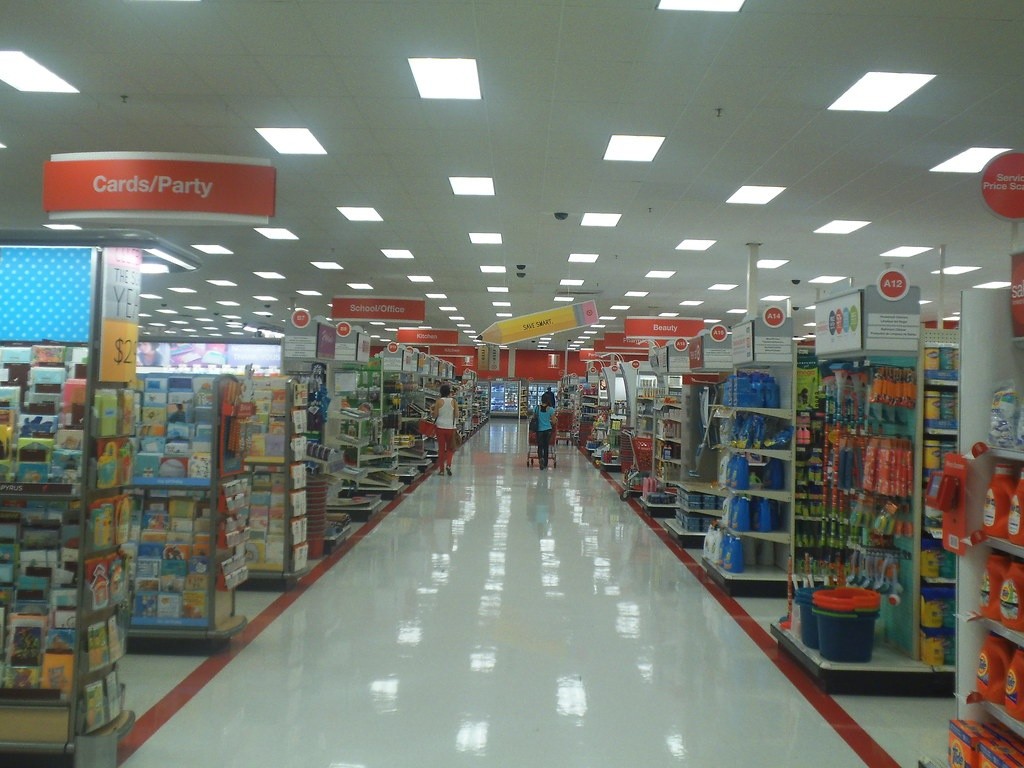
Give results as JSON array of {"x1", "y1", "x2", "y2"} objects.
[
  {"x1": 1007, "y1": 467, "x2": 1024, "y2": 546},
  {"x1": 999, "y1": 557, "x2": 1024, "y2": 632},
  {"x1": 983, "y1": 464, "x2": 1016, "y2": 539},
  {"x1": 719, "y1": 451, "x2": 749, "y2": 490},
  {"x1": 976, "y1": 630, "x2": 1010, "y2": 705},
  {"x1": 722, "y1": 493, "x2": 779, "y2": 532},
  {"x1": 723, "y1": 372, "x2": 779, "y2": 407},
  {"x1": 704, "y1": 519, "x2": 774, "y2": 573},
  {"x1": 1005, "y1": 645, "x2": 1024, "y2": 722},
  {"x1": 762, "y1": 458, "x2": 784, "y2": 489},
  {"x1": 986, "y1": 378, "x2": 1024, "y2": 451},
  {"x1": 978, "y1": 548, "x2": 1010, "y2": 620},
  {"x1": 720, "y1": 413, "x2": 794, "y2": 449}
]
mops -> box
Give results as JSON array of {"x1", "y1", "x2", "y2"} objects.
[{"x1": 829, "y1": 363, "x2": 866, "y2": 550}]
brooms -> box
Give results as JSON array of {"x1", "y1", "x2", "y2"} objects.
[{"x1": 818, "y1": 358, "x2": 867, "y2": 546}]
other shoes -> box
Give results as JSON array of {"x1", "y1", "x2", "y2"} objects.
[
  {"x1": 437, "y1": 471, "x2": 444, "y2": 476},
  {"x1": 445, "y1": 465, "x2": 452, "y2": 476},
  {"x1": 539, "y1": 459, "x2": 547, "y2": 470}
]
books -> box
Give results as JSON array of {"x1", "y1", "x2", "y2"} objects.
[{"x1": 0, "y1": 344, "x2": 286, "y2": 731}]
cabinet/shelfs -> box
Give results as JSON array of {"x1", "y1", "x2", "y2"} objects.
[
  {"x1": 324, "y1": 381, "x2": 489, "y2": 523},
  {"x1": 707, "y1": 404, "x2": 791, "y2": 580},
  {"x1": 667, "y1": 479, "x2": 726, "y2": 539},
  {"x1": 978, "y1": 445, "x2": 1024, "y2": 739},
  {"x1": 0, "y1": 253, "x2": 308, "y2": 768},
  {"x1": 623, "y1": 398, "x2": 654, "y2": 487},
  {"x1": 641, "y1": 403, "x2": 682, "y2": 509},
  {"x1": 918, "y1": 342, "x2": 956, "y2": 663},
  {"x1": 575, "y1": 396, "x2": 597, "y2": 440}
]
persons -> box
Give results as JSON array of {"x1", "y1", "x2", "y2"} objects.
[
  {"x1": 543, "y1": 386, "x2": 554, "y2": 406},
  {"x1": 433, "y1": 385, "x2": 460, "y2": 477},
  {"x1": 533, "y1": 395, "x2": 556, "y2": 471}
]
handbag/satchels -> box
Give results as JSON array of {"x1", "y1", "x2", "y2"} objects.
[
  {"x1": 529, "y1": 405, "x2": 539, "y2": 431},
  {"x1": 450, "y1": 430, "x2": 460, "y2": 451}
]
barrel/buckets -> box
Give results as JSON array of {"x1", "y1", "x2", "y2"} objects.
[{"x1": 795, "y1": 587, "x2": 883, "y2": 662}]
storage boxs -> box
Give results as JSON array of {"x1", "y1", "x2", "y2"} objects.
[{"x1": 621, "y1": 436, "x2": 725, "y2": 532}]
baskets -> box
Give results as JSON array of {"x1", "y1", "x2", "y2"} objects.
[
  {"x1": 620, "y1": 436, "x2": 634, "y2": 473},
  {"x1": 418, "y1": 413, "x2": 438, "y2": 438}
]
